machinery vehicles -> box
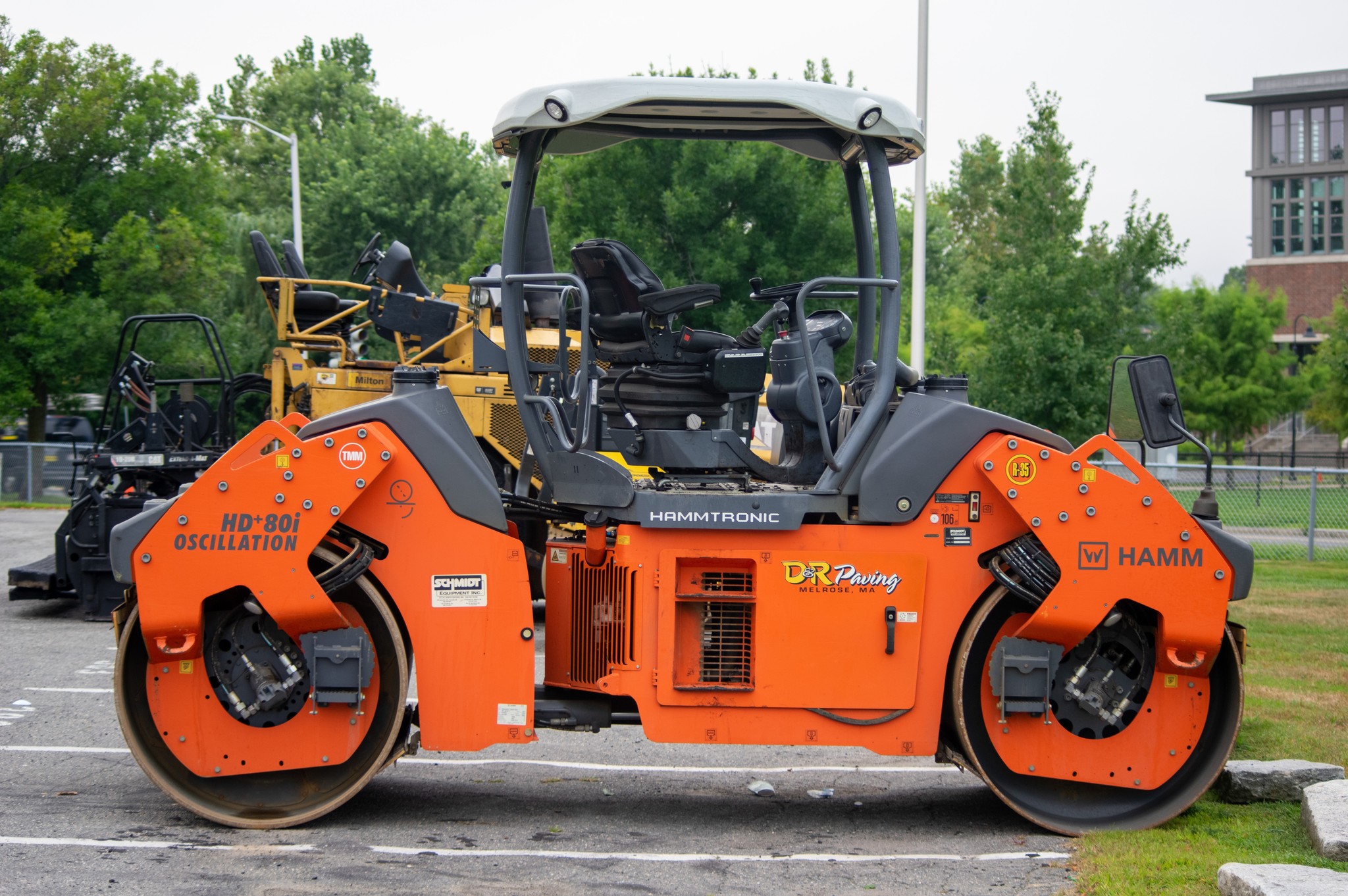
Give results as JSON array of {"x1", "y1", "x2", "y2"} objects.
[
  {"x1": 8, "y1": 233, "x2": 849, "y2": 625},
  {"x1": 108, "y1": 75, "x2": 1257, "y2": 840}
]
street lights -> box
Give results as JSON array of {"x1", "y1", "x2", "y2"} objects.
[
  {"x1": 1289, "y1": 313, "x2": 1316, "y2": 480},
  {"x1": 215, "y1": 113, "x2": 305, "y2": 267}
]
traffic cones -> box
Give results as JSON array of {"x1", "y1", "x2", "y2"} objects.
[{"x1": 1316, "y1": 473, "x2": 1323, "y2": 482}]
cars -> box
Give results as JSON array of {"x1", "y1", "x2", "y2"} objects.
[{"x1": 0, "y1": 393, "x2": 106, "y2": 494}]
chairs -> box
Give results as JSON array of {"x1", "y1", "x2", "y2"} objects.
[
  {"x1": 281, "y1": 237, "x2": 359, "y2": 314},
  {"x1": 249, "y1": 229, "x2": 341, "y2": 316},
  {"x1": 570, "y1": 239, "x2": 739, "y2": 363}
]
